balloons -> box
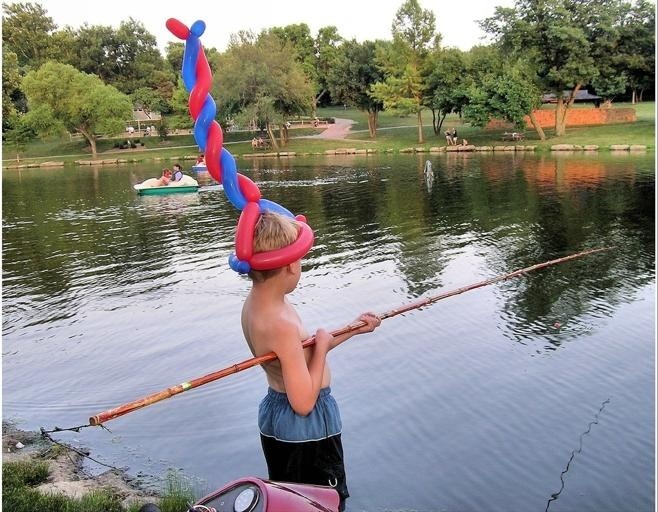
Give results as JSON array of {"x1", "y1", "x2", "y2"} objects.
[{"x1": 165, "y1": 18, "x2": 314, "y2": 274}]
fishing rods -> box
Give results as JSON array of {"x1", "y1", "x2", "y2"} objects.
[{"x1": 88, "y1": 246, "x2": 620, "y2": 428}]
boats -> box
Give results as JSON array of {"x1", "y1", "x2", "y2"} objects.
[
  {"x1": 191, "y1": 164, "x2": 211, "y2": 175},
  {"x1": 133, "y1": 174, "x2": 201, "y2": 197}
]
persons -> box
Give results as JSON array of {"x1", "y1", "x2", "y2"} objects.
[
  {"x1": 445, "y1": 128, "x2": 453, "y2": 146},
  {"x1": 151, "y1": 164, "x2": 183, "y2": 188},
  {"x1": 128, "y1": 127, "x2": 134, "y2": 136},
  {"x1": 240, "y1": 208, "x2": 382, "y2": 512},
  {"x1": 151, "y1": 124, "x2": 155, "y2": 131},
  {"x1": 162, "y1": 168, "x2": 172, "y2": 178},
  {"x1": 451, "y1": 128, "x2": 457, "y2": 146},
  {"x1": 144, "y1": 126, "x2": 150, "y2": 136}
]
